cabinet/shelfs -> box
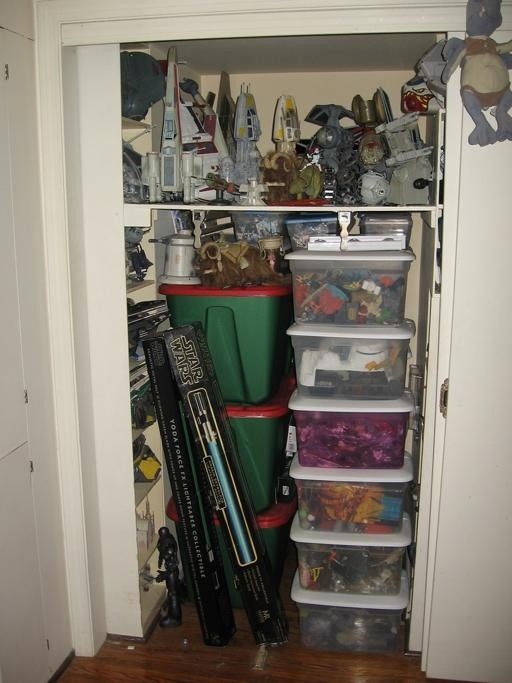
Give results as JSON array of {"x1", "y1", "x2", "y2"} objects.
[{"x1": 76, "y1": 39, "x2": 166, "y2": 642}]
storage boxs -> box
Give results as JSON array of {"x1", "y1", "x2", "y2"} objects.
[
  {"x1": 158, "y1": 275, "x2": 289, "y2": 404},
  {"x1": 282, "y1": 210, "x2": 417, "y2": 655},
  {"x1": 221, "y1": 377, "x2": 299, "y2": 515},
  {"x1": 166, "y1": 492, "x2": 298, "y2": 608},
  {"x1": 229, "y1": 211, "x2": 288, "y2": 271}
]
